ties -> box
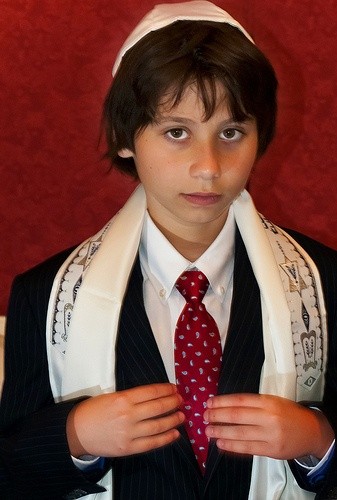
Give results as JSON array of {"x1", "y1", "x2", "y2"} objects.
[{"x1": 174, "y1": 267, "x2": 223, "y2": 476}]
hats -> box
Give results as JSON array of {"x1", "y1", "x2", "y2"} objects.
[{"x1": 112, "y1": 0, "x2": 255, "y2": 78}]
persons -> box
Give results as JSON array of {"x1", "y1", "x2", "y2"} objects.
[{"x1": 0, "y1": 0, "x2": 337, "y2": 500}]
